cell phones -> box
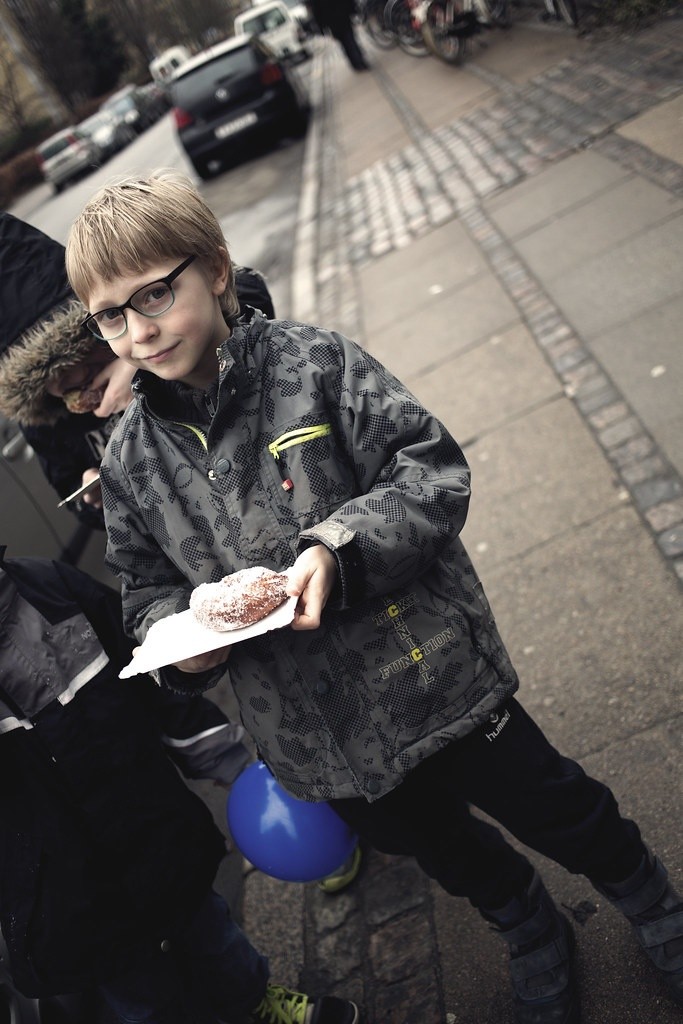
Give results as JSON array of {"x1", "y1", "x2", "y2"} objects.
[{"x1": 57, "y1": 476, "x2": 101, "y2": 510}]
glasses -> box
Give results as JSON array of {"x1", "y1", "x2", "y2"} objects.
[{"x1": 81, "y1": 254, "x2": 199, "y2": 340}]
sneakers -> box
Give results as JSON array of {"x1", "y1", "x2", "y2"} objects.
[
  {"x1": 250, "y1": 984, "x2": 358, "y2": 1024},
  {"x1": 316, "y1": 828, "x2": 361, "y2": 892}
]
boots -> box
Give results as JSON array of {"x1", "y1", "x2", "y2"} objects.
[
  {"x1": 478, "y1": 869, "x2": 582, "y2": 1024},
  {"x1": 591, "y1": 838, "x2": 683, "y2": 1002}
]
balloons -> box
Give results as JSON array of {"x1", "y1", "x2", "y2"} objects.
[{"x1": 219, "y1": 751, "x2": 376, "y2": 898}]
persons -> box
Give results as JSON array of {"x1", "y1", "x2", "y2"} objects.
[
  {"x1": 306, "y1": 0, "x2": 375, "y2": 76},
  {"x1": 0, "y1": 170, "x2": 682, "y2": 1024}
]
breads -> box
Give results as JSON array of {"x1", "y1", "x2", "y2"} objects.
[
  {"x1": 62, "y1": 381, "x2": 107, "y2": 414},
  {"x1": 188, "y1": 566, "x2": 289, "y2": 631}
]
